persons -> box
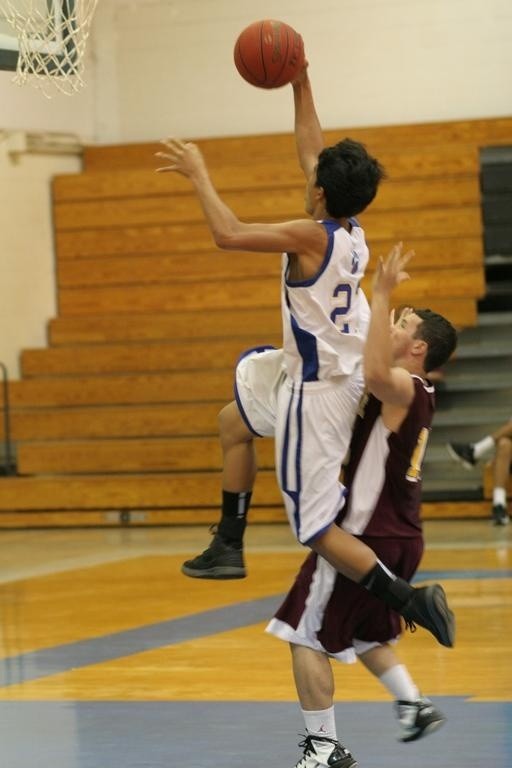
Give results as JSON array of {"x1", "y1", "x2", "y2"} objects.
[
  {"x1": 446, "y1": 410, "x2": 512, "y2": 526},
  {"x1": 273, "y1": 240, "x2": 460, "y2": 768},
  {"x1": 152, "y1": 61, "x2": 456, "y2": 649}
]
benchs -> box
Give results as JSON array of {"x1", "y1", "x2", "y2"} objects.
[{"x1": 0, "y1": 117, "x2": 512, "y2": 531}]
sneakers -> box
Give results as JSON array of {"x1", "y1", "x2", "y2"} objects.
[
  {"x1": 181, "y1": 535, "x2": 245, "y2": 580},
  {"x1": 447, "y1": 441, "x2": 476, "y2": 469},
  {"x1": 493, "y1": 504, "x2": 509, "y2": 526},
  {"x1": 295, "y1": 735, "x2": 357, "y2": 767},
  {"x1": 396, "y1": 700, "x2": 443, "y2": 742},
  {"x1": 405, "y1": 584, "x2": 454, "y2": 648}
]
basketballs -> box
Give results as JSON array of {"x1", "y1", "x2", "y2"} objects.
[{"x1": 234, "y1": 21, "x2": 304, "y2": 89}]
